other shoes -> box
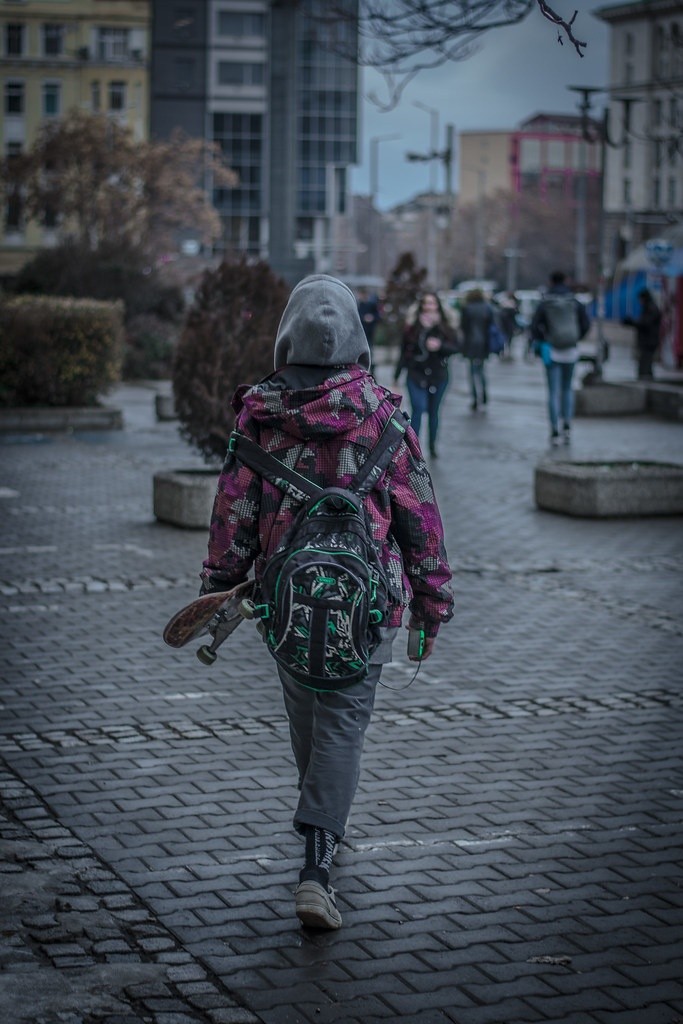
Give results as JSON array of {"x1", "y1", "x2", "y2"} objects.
[
  {"x1": 552, "y1": 436, "x2": 559, "y2": 445},
  {"x1": 430, "y1": 444, "x2": 437, "y2": 458},
  {"x1": 564, "y1": 436, "x2": 570, "y2": 444}
]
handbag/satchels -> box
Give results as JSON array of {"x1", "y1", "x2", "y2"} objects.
[{"x1": 489, "y1": 325, "x2": 505, "y2": 352}]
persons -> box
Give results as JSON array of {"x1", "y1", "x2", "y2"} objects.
[
  {"x1": 199, "y1": 271, "x2": 453, "y2": 930},
  {"x1": 622, "y1": 288, "x2": 662, "y2": 382},
  {"x1": 530, "y1": 271, "x2": 590, "y2": 446},
  {"x1": 394, "y1": 290, "x2": 460, "y2": 460},
  {"x1": 457, "y1": 288, "x2": 495, "y2": 409},
  {"x1": 353, "y1": 274, "x2": 683, "y2": 374}
]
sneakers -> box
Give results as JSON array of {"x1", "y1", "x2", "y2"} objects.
[{"x1": 291, "y1": 880, "x2": 342, "y2": 929}]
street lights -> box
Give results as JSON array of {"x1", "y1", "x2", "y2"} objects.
[{"x1": 368, "y1": 99, "x2": 438, "y2": 293}]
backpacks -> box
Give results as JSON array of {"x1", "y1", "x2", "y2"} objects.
[
  {"x1": 547, "y1": 299, "x2": 579, "y2": 349},
  {"x1": 227, "y1": 410, "x2": 410, "y2": 691}
]
